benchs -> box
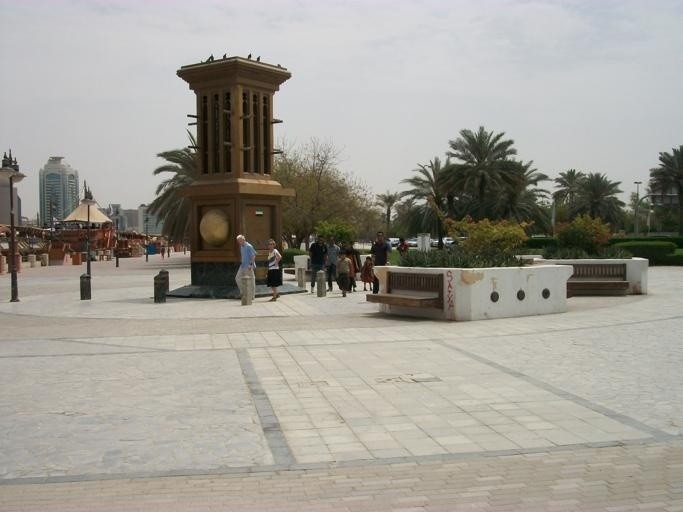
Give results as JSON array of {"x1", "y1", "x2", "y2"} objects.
[
  {"x1": 556, "y1": 264, "x2": 629, "y2": 289},
  {"x1": 366, "y1": 272, "x2": 444, "y2": 313}
]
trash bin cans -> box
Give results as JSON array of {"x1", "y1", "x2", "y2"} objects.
[
  {"x1": 8, "y1": 254, "x2": 22, "y2": 272},
  {"x1": 293, "y1": 255, "x2": 310, "y2": 280},
  {"x1": 72, "y1": 252, "x2": 81, "y2": 265}
]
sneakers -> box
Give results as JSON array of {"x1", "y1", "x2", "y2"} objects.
[
  {"x1": 310, "y1": 288, "x2": 372, "y2": 297},
  {"x1": 269, "y1": 294, "x2": 280, "y2": 302}
]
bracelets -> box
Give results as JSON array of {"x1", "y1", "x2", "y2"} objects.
[{"x1": 249, "y1": 263, "x2": 252, "y2": 266}]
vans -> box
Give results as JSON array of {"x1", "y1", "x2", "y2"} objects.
[{"x1": 388, "y1": 237, "x2": 399, "y2": 246}]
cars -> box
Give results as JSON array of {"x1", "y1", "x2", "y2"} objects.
[{"x1": 407, "y1": 236, "x2": 467, "y2": 247}]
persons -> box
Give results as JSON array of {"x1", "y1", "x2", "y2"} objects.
[
  {"x1": 233, "y1": 233, "x2": 255, "y2": 300},
  {"x1": 326, "y1": 231, "x2": 409, "y2": 297},
  {"x1": 308, "y1": 234, "x2": 327, "y2": 293},
  {"x1": 265, "y1": 239, "x2": 283, "y2": 301}
]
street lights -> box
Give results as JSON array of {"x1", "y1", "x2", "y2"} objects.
[
  {"x1": 0, "y1": 148, "x2": 26, "y2": 302},
  {"x1": 634, "y1": 179, "x2": 642, "y2": 237}
]
